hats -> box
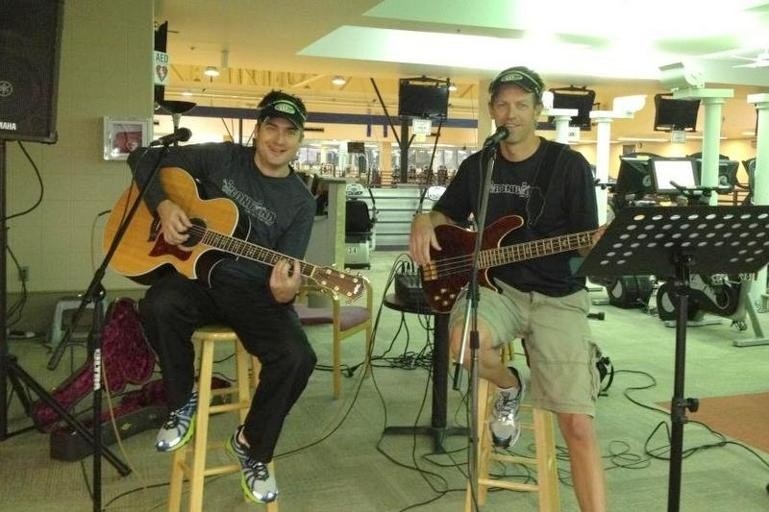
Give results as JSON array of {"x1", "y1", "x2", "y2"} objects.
[
  {"x1": 259, "y1": 100, "x2": 305, "y2": 131},
  {"x1": 490, "y1": 69, "x2": 543, "y2": 101}
]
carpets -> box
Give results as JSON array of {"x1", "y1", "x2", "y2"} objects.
[{"x1": 655, "y1": 390, "x2": 768, "y2": 455}]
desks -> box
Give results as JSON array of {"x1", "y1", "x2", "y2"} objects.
[{"x1": 383, "y1": 294, "x2": 470, "y2": 453}]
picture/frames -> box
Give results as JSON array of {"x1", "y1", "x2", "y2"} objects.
[{"x1": 102, "y1": 118, "x2": 153, "y2": 161}]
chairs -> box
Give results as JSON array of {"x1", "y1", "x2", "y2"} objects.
[{"x1": 292, "y1": 257, "x2": 375, "y2": 400}]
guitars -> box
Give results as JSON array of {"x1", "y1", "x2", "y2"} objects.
[
  {"x1": 102, "y1": 167, "x2": 366, "y2": 305},
  {"x1": 419, "y1": 215, "x2": 609, "y2": 314}
]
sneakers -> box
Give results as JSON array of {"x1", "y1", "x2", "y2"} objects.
[
  {"x1": 155, "y1": 381, "x2": 212, "y2": 452},
  {"x1": 487, "y1": 367, "x2": 526, "y2": 449},
  {"x1": 225, "y1": 426, "x2": 279, "y2": 504}
]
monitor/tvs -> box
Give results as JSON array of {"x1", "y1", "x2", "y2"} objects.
[
  {"x1": 397, "y1": 84, "x2": 449, "y2": 123},
  {"x1": 653, "y1": 98, "x2": 700, "y2": 133},
  {"x1": 548, "y1": 92, "x2": 594, "y2": 127},
  {"x1": 648, "y1": 157, "x2": 700, "y2": 202}
]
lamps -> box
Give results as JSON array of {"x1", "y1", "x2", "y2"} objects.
[
  {"x1": 203, "y1": 66, "x2": 219, "y2": 76},
  {"x1": 331, "y1": 75, "x2": 346, "y2": 86}
]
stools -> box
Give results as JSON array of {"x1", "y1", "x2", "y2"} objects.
[
  {"x1": 166, "y1": 325, "x2": 282, "y2": 511},
  {"x1": 51, "y1": 294, "x2": 112, "y2": 346},
  {"x1": 464, "y1": 331, "x2": 563, "y2": 512}
]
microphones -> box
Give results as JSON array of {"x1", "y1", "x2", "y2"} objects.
[
  {"x1": 484, "y1": 126, "x2": 510, "y2": 146},
  {"x1": 149, "y1": 127, "x2": 192, "y2": 147}
]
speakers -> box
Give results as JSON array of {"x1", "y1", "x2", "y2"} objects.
[{"x1": 0, "y1": 0, "x2": 65, "y2": 145}]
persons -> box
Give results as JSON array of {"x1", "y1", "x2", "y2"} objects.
[
  {"x1": 126, "y1": 88, "x2": 318, "y2": 503},
  {"x1": 407, "y1": 67, "x2": 608, "y2": 512}
]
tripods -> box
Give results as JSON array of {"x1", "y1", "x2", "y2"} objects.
[{"x1": 0, "y1": 138, "x2": 134, "y2": 480}]
